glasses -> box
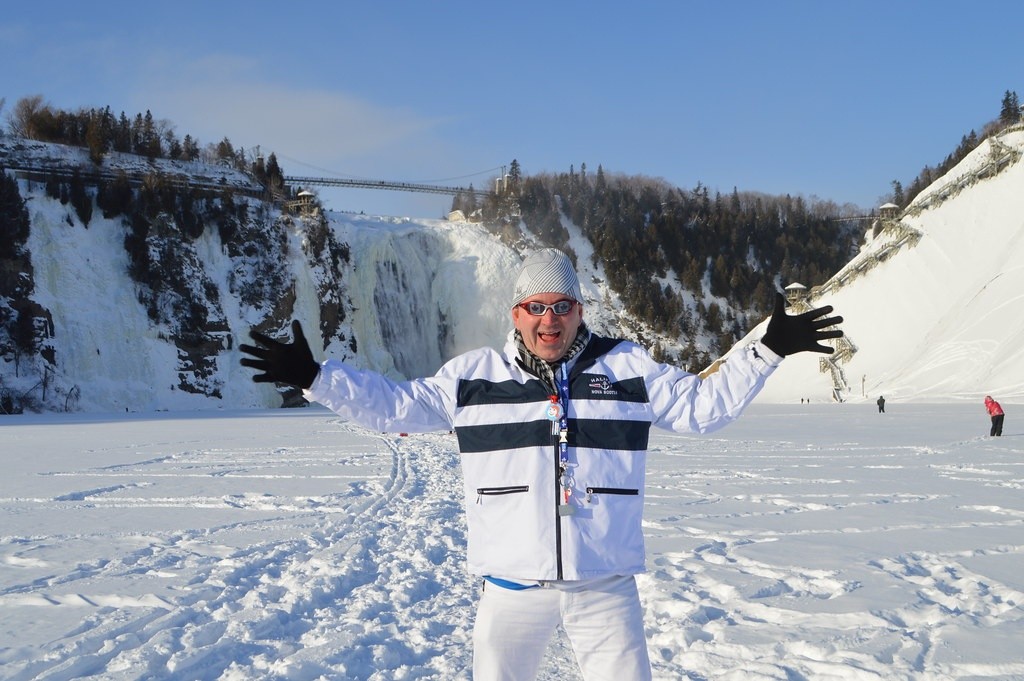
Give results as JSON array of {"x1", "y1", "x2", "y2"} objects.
[{"x1": 520, "y1": 300, "x2": 576, "y2": 316}]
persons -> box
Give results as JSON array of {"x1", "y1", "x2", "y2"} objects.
[
  {"x1": 877, "y1": 396, "x2": 885, "y2": 413},
  {"x1": 239, "y1": 248, "x2": 844, "y2": 681},
  {"x1": 984, "y1": 396, "x2": 1005, "y2": 436},
  {"x1": 807, "y1": 398, "x2": 810, "y2": 404},
  {"x1": 800, "y1": 398, "x2": 804, "y2": 404}
]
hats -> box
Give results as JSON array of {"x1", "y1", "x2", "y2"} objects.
[{"x1": 512, "y1": 249, "x2": 583, "y2": 309}]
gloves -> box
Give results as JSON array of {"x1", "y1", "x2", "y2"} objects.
[
  {"x1": 238, "y1": 320, "x2": 321, "y2": 388},
  {"x1": 760, "y1": 294, "x2": 844, "y2": 356}
]
